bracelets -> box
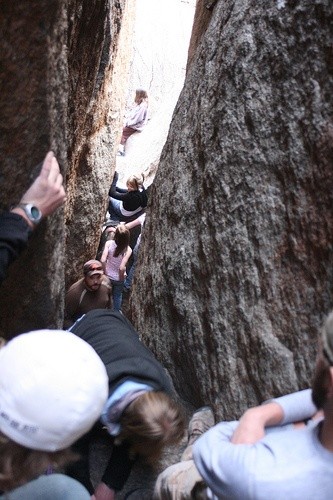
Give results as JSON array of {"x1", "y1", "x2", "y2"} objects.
[{"x1": 304, "y1": 417, "x2": 313, "y2": 427}]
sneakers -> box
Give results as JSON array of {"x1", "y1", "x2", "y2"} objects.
[{"x1": 181, "y1": 406, "x2": 215, "y2": 463}]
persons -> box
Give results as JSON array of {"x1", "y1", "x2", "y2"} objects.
[
  {"x1": 155, "y1": 314, "x2": 333, "y2": 500},
  {"x1": 117, "y1": 89, "x2": 149, "y2": 156},
  {"x1": 0, "y1": 150, "x2": 187, "y2": 500}
]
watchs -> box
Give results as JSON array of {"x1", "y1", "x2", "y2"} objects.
[{"x1": 14, "y1": 203, "x2": 41, "y2": 225}]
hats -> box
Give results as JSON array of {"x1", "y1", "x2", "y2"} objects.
[
  {"x1": 83, "y1": 259, "x2": 103, "y2": 277},
  {"x1": 0, "y1": 329, "x2": 109, "y2": 452},
  {"x1": 322, "y1": 311, "x2": 333, "y2": 366}
]
